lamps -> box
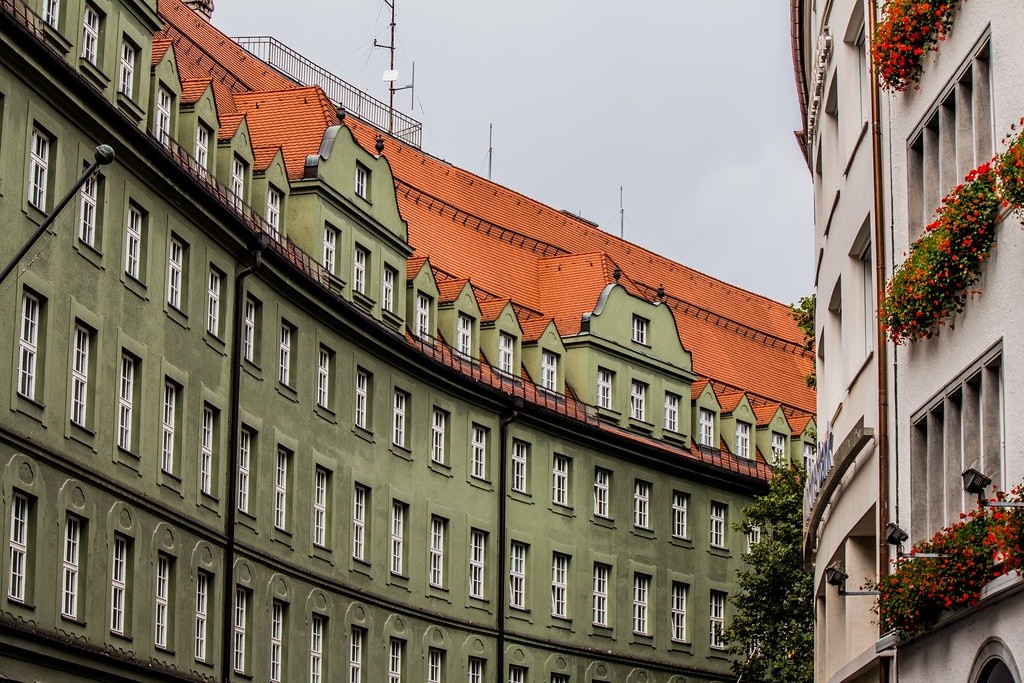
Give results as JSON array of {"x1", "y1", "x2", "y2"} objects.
[
  {"x1": 961, "y1": 469, "x2": 1024, "y2": 507},
  {"x1": 884, "y1": 522, "x2": 948, "y2": 558},
  {"x1": 825, "y1": 566, "x2": 880, "y2": 596}
]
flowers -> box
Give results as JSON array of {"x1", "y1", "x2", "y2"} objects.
[
  {"x1": 875, "y1": 161, "x2": 1003, "y2": 346},
  {"x1": 991, "y1": 117, "x2": 1024, "y2": 230},
  {"x1": 864, "y1": 0, "x2": 967, "y2": 97},
  {"x1": 859, "y1": 477, "x2": 1024, "y2": 649}
]
